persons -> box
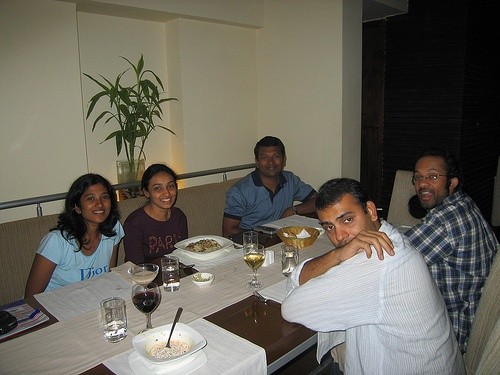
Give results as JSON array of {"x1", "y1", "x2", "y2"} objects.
[
  {"x1": 403, "y1": 149, "x2": 500, "y2": 354},
  {"x1": 222, "y1": 136, "x2": 318, "y2": 238},
  {"x1": 123, "y1": 164, "x2": 188, "y2": 265},
  {"x1": 281, "y1": 178, "x2": 468, "y2": 375},
  {"x1": 24, "y1": 173, "x2": 125, "y2": 297}
]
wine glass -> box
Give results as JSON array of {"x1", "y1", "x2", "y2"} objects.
[
  {"x1": 131, "y1": 281, "x2": 162, "y2": 335},
  {"x1": 244, "y1": 244, "x2": 266, "y2": 288}
]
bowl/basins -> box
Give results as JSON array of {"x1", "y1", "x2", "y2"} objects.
[
  {"x1": 174, "y1": 234, "x2": 234, "y2": 258},
  {"x1": 132, "y1": 322, "x2": 207, "y2": 364},
  {"x1": 276, "y1": 226, "x2": 319, "y2": 248},
  {"x1": 190, "y1": 272, "x2": 214, "y2": 286}
]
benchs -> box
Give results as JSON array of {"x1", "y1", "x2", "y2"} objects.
[{"x1": 0, "y1": 160, "x2": 261, "y2": 304}]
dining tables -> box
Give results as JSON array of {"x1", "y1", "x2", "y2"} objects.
[{"x1": 0, "y1": 209, "x2": 413, "y2": 375}]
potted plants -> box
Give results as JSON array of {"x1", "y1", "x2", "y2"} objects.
[{"x1": 79, "y1": 52, "x2": 179, "y2": 202}]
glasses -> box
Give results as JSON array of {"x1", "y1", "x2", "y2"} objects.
[{"x1": 410, "y1": 174, "x2": 448, "y2": 184}]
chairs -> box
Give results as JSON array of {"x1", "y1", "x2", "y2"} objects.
[
  {"x1": 387, "y1": 171, "x2": 423, "y2": 227},
  {"x1": 461, "y1": 250, "x2": 500, "y2": 375}
]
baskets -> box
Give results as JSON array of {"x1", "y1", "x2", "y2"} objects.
[{"x1": 277, "y1": 226, "x2": 320, "y2": 248}]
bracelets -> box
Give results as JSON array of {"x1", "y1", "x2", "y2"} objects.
[{"x1": 289, "y1": 206, "x2": 297, "y2": 214}]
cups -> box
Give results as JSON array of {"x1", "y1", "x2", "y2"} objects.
[
  {"x1": 161, "y1": 257, "x2": 180, "y2": 292},
  {"x1": 281, "y1": 245, "x2": 300, "y2": 275},
  {"x1": 100, "y1": 297, "x2": 128, "y2": 344},
  {"x1": 242, "y1": 232, "x2": 259, "y2": 251},
  {"x1": 127, "y1": 263, "x2": 159, "y2": 285}
]
